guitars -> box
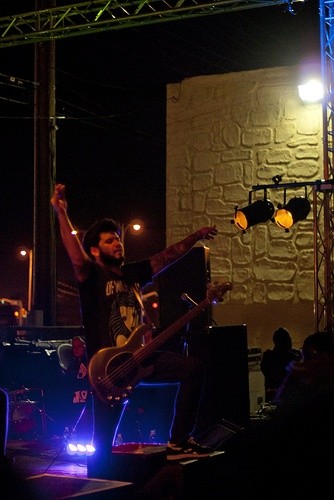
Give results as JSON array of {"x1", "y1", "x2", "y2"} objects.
[{"x1": 89, "y1": 280, "x2": 233, "y2": 409}]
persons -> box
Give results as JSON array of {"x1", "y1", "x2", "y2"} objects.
[
  {"x1": 51, "y1": 184, "x2": 218, "y2": 481},
  {"x1": 260, "y1": 327, "x2": 333, "y2": 500}
]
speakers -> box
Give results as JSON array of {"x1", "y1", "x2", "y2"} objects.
[
  {"x1": 153, "y1": 245, "x2": 211, "y2": 328},
  {"x1": 173, "y1": 324, "x2": 249, "y2": 439}
]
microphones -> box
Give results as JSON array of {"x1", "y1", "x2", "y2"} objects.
[{"x1": 179, "y1": 293, "x2": 199, "y2": 307}]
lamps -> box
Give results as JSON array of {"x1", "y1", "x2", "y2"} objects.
[
  {"x1": 272, "y1": 197, "x2": 311, "y2": 233},
  {"x1": 231, "y1": 199, "x2": 274, "y2": 234}
]
